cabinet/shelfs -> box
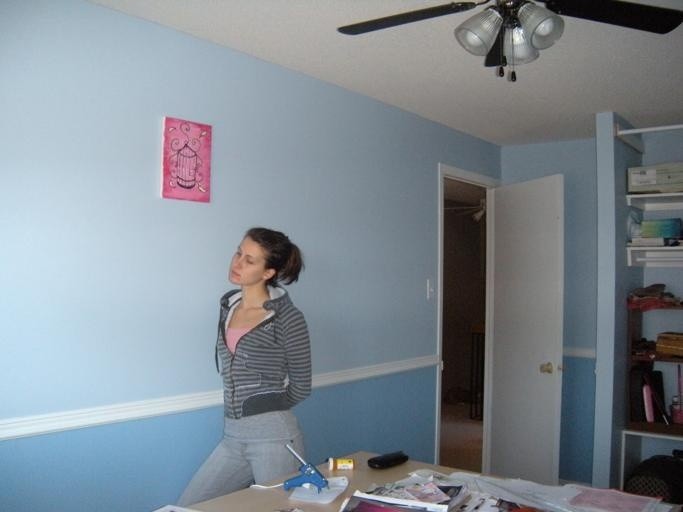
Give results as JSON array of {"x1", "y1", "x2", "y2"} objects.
[
  {"x1": 625, "y1": 296, "x2": 683, "y2": 437},
  {"x1": 624, "y1": 192, "x2": 683, "y2": 268}
]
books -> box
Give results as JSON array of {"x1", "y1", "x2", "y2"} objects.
[{"x1": 340, "y1": 484, "x2": 484, "y2": 512}]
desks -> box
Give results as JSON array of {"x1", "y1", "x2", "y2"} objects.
[{"x1": 168, "y1": 450, "x2": 683, "y2": 512}]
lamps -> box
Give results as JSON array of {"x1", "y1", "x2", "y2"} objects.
[{"x1": 454, "y1": 0, "x2": 565, "y2": 67}]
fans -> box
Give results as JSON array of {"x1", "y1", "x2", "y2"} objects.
[{"x1": 335, "y1": 0, "x2": 683, "y2": 38}]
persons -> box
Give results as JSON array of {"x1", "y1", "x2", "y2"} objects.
[{"x1": 177, "y1": 229, "x2": 312, "y2": 508}]
